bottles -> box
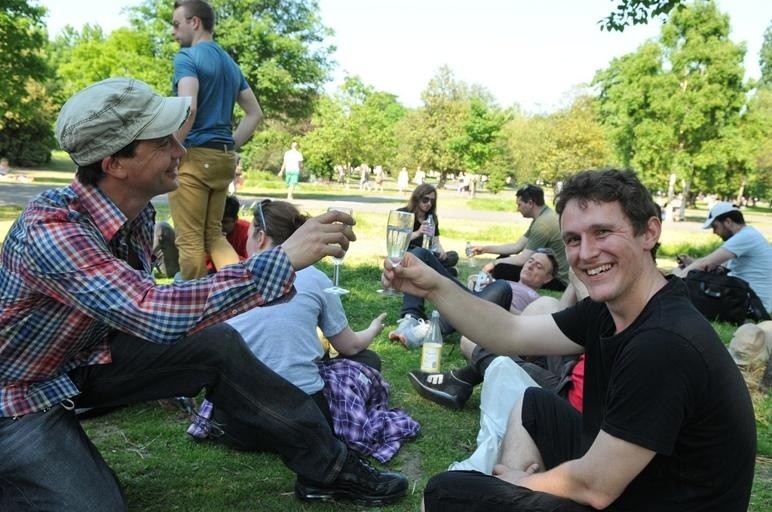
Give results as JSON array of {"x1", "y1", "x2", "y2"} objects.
[
  {"x1": 421, "y1": 309, "x2": 443, "y2": 371},
  {"x1": 423, "y1": 214, "x2": 435, "y2": 250},
  {"x1": 467, "y1": 242, "x2": 477, "y2": 267}
]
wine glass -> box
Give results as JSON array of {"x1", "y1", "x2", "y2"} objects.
[
  {"x1": 376, "y1": 209, "x2": 414, "y2": 298},
  {"x1": 323, "y1": 207, "x2": 351, "y2": 295}
]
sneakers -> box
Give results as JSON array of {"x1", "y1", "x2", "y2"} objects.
[
  {"x1": 388, "y1": 314, "x2": 431, "y2": 352},
  {"x1": 407, "y1": 369, "x2": 474, "y2": 412},
  {"x1": 296, "y1": 445, "x2": 409, "y2": 507}
]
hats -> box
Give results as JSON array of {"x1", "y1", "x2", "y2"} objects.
[
  {"x1": 728, "y1": 322, "x2": 766, "y2": 366},
  {"x1": 55, "y1": 76, "x2": 193, "y2": 166},
  {"x1": 702, "y1": 201, "x2": 740, "y2": 229}
]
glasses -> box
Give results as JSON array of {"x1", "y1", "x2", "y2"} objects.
[
  {"x1": 422, "y1": 197, "x2": 435, "y2": 206},
  {"x1": 172, "y1": 15, "x2": 196, "y2": 29},
  {"x1": 253, "y1": 199, "x2": 273, "y2": 235}
]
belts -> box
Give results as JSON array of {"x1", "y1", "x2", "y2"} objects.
[{"x1": 199, "y1": 140, "x2": 234, "y2": 152}]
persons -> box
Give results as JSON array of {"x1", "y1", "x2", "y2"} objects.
[
  {"x1": 338, "y1": 163, "x2": 426, "y2": 193},
  {"x1": 729, "y1": 321, "x2": 772, "y2": 393},
  {"x1": 465, "y1": 185, "x2": 570, "y2": 292},
  {"x1": 0, "y1": 77, "x2": 409, "y2": 510},
  {"x1": 409, "y1": 202, "x2": 661, "y2": 416},
  {"x1": 227, "y1": 154, "x2": 241, "y2": 195},
  {"x1": 389, "y1": 246, "x2": 559, "y2": 352},
  {"x1": 166, "y1": 0, "x2": 265, "y2": 281},
  {"x1": 381, "y1": 169, "x2": 757, "y2": 512},
  {"x1": 277, "y1": 141, "x2": 304, "y2": 200},
  {"x1": 398, "y1": 184, "x2": 459, "y2": 279},
  {"x1": 153, "y1": 193, "x2": 421, "y2": 464},
  {"x1": 666, "y1": 201, "x2": 772, "y2": 323}
]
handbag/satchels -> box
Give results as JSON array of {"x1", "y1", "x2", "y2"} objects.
[{"x1": 685, "y1": 269, "x2": 751, "y2": 327}]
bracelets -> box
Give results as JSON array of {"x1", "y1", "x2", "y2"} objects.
[{"x1": 490, "y1": 261, "x2": 495, "y2": 267}]
